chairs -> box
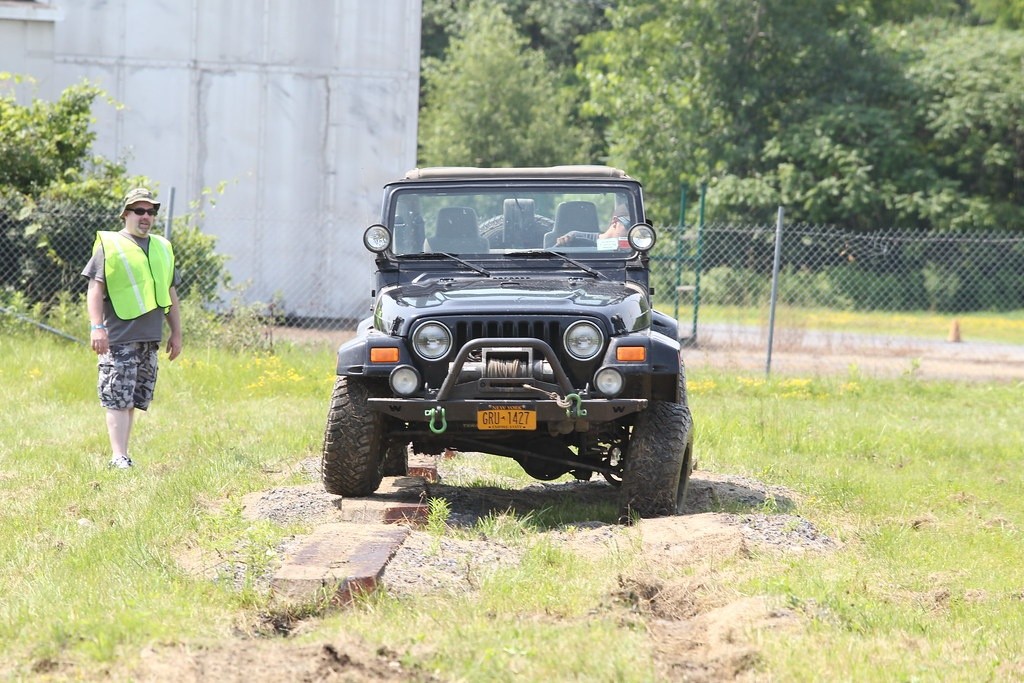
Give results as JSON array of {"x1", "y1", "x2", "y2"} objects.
[
  {"x1": 542, "y1": 200, "x2": 604, "y2": 251},
  {"x1": 423, "y1": 206, "x2": 491, "y2": 257}
]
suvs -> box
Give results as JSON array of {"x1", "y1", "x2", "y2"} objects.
[{"x1": 319, "y1": 163, "x2": 693, "y2": 521}]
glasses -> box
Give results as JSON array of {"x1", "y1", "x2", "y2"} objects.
[
  {"x1": 619, "y1": 217, "x2": 632, "y2": 230},
  {"x1": 127, "y1": 207, "x2": 156, "y2": 215}
]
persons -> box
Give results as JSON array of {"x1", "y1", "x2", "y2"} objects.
[
  {"x1": 82, "y1": 188, "x2": 182, "y2": 470},
  {"x1": 557, "y1": 201, "x2": 653, "y2": 246}
]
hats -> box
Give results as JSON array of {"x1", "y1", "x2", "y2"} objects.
[
  {"x1": 119, "y1": 188, "x2": 161, "y2": 219},
  {"x1": 614, "y1": 203, "x2": 653, "y2": 226}
]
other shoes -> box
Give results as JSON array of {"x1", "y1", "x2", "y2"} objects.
[{"x1": 111, "y1": 456, "x2": 134, "y2": 470}]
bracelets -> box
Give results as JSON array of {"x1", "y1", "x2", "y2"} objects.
[{"x1": 92, "y1": 324, "x2": 104, "y2": 329}]
